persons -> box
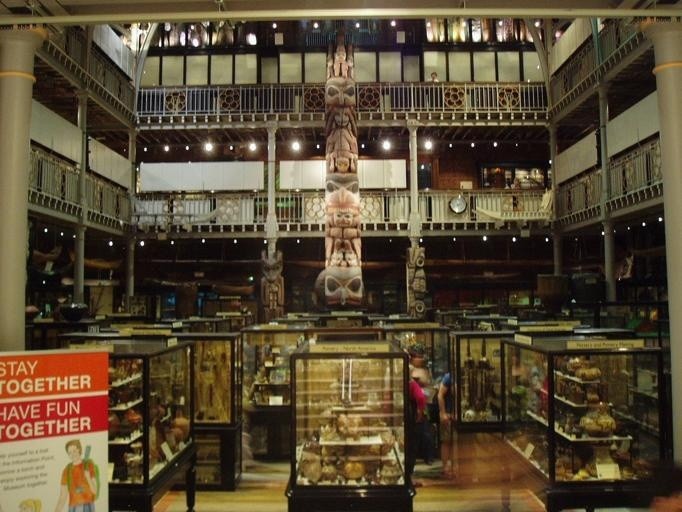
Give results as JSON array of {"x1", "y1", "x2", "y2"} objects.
[
  {"x1": 404, "y1": 341, "x2": 451, "y2": 488},
  {"x1": 53, "y1": 439, "x2": 99, "y2": 512},
  {"x1": 511, "y1": 178, "x2": 522, "y2": 189},
  {"x1": 511, "y1": 350, "x2": 548, "y2": 442},
  {"x1": 426, "y1": 72, "x2": 439, "y2": 83},
  {"x1": 18, "y1": 499, "x2": 44, "y2": 511}
]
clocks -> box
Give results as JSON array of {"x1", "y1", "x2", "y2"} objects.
[{"x1": 449, "y1": 195, "x2": 467, "y2": 213}]
